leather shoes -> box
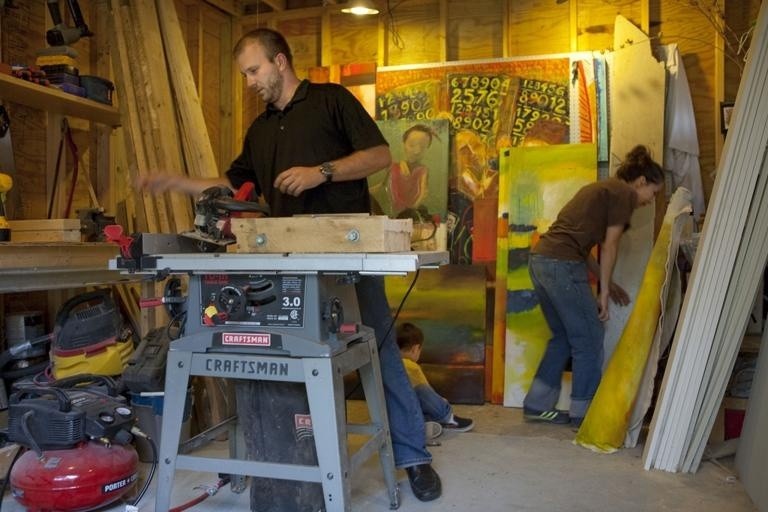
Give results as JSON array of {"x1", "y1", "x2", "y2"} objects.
[{"x1": 404, "y1": 462, "x2": 442, "y2": 502}]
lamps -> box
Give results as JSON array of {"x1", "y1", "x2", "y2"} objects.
[{"x1": 340, "y1": 1, "x2": 379, "y2": 16}]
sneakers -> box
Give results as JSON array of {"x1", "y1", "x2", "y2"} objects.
[
  {"x1": 571, "y1": 418, "x2": 582, "y2": 431},
  {"x1": 522, "y1": 407, "x2": 570, "y2": 424},
  {"x1": 421, "y1": 421, "x2": 441, "y2": 439},
  {"x1": 441, "y1": 415, "x2": 474, "y2": 433}
]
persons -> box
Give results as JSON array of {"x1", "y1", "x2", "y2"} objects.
[
  {"x1": 395, "y1": 322, "x2": 475, "y2": 440},
  {"x1": 522, "y1": 143, "x2": 668, "y2": 432},
  {"x1": 390, "y1": 124, "x2": 493, "y2": 211},
  {"x1": 137, "y1": 29, "x2": 444, "y2": 502}
]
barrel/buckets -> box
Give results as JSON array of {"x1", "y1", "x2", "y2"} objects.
[{"x1": 128, "y1": 390, "x2": 192, "y2": 463}]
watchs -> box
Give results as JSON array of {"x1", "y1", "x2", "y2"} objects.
[{"x1": 319, "y1": 161, "x2": 336, "y2": 185}]
